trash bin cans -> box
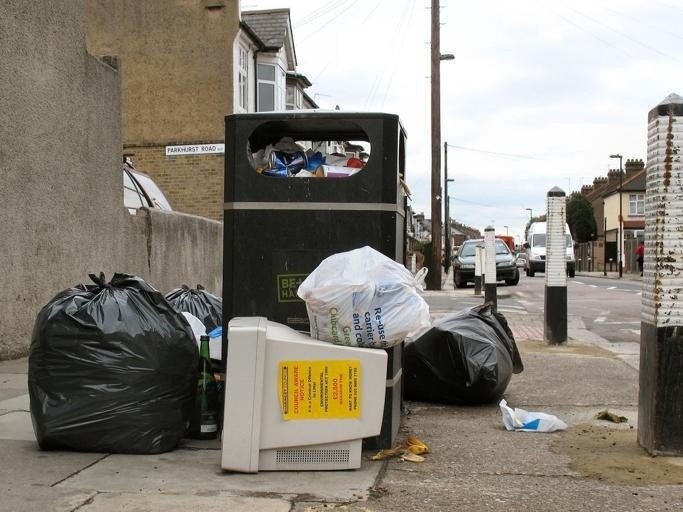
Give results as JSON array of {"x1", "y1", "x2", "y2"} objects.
[{"x1": 220, "y1": 112, "x2": 409, "y2": 452}]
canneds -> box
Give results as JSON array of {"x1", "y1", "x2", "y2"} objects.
[{"x1": 260, "y1": 149, "x2": 362, "y2": 179}]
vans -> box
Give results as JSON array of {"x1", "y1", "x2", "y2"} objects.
[{"x1": 523, "y1": 222, "x2": 576, "y2": 277}]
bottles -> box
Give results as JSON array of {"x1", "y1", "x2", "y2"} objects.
[{"x1": 191, "y1": 335, "x2": 218, "y2": 440}]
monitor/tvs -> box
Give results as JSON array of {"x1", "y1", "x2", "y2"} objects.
[{"x1": 220, "y1": 316, "x2": 388, "y2": 473}]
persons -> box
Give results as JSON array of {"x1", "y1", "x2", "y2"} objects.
[{"x1": 633, "y1": 241, "x2": 643, "y2": 277}]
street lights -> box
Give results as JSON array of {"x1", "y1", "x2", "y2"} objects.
[{"x1": 609, "y1": 154, "x2": 622, "y2": 278}]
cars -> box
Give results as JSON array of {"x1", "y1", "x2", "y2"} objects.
[{"x1": 452, "y1": 238, "x2": 526, "y2": 288}]
